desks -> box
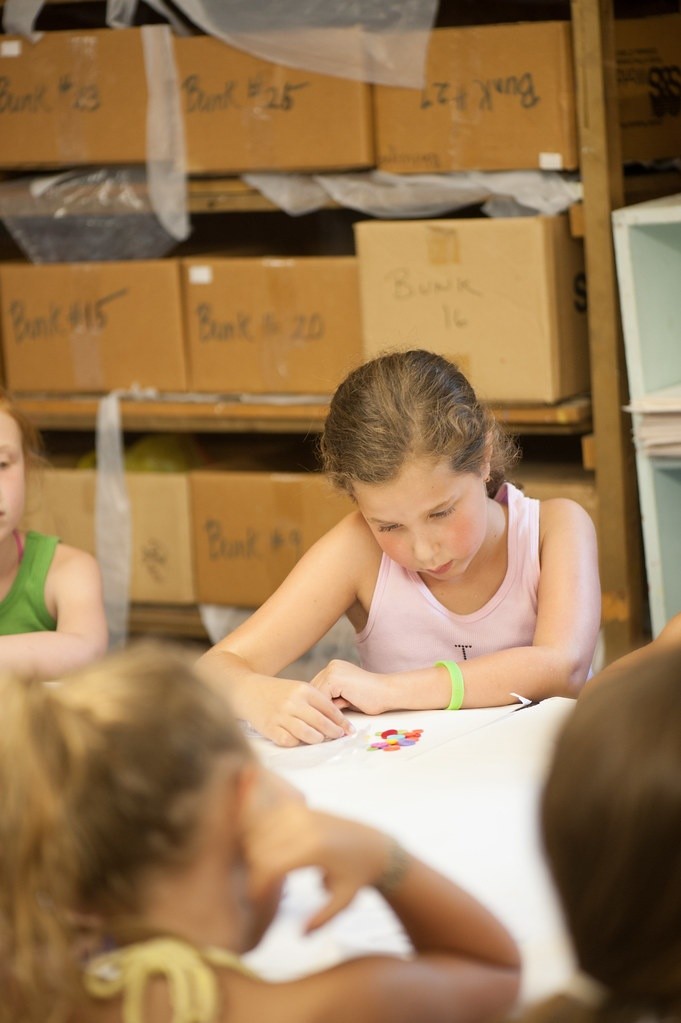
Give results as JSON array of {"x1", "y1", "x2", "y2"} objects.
[{"x1": 233, "y1": 698, "x2": 581, "y2": 1003}]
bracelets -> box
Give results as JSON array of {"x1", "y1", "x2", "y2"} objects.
[
  {"x1": 369, "y1": 830, "x2": 403, "y2": 894},
  {"x1": 433, "y1": 659, "x2": 465, "y2": 713}
]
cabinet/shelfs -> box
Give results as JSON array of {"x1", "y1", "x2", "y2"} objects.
[
  {"x1": 0, "y1": 0, "x2": 681, "y2": 668},
  {"x1": 611, "y1": 194, "x2": 681, "y2": 640}
]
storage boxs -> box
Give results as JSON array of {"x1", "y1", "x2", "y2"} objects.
[
  {"x1": 0, "y1": 215, "x2": 587, "y2": 409},
  {"x1": 0, "y1": 16, "x2": 681, "y2": 181},
  {"x1": 28, "y1": 456, "x2": 596, "y2": 609}
]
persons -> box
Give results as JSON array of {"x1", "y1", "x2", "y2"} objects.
[
  {"x1": 1, "y1": 637, "x2": 521, "y2": 1021},
  {"x1": 535, "y1": 644, "x2": 680, "y2": 1022},
  {"x1": 1, "y1": 382, "x2": 109, "y2": 680},
  {"x1": 574, "y1": 610, "x2": 680, "y2": 705},
  {"x1": 176, "y1": 350, "x2": 608, "y2": 748}
]
